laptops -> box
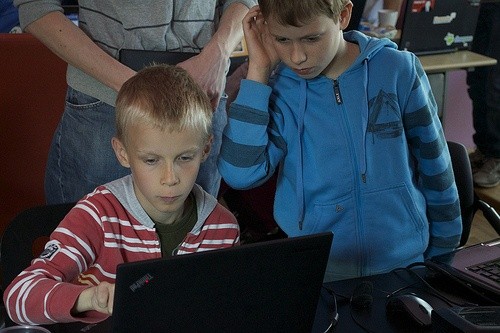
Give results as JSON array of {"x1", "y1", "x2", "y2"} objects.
[
  {"x1": 111, "y1": 231, "x2": 335, "y2": 333},
  {"x1": 398, "y1": 0, "x2": 480, "y2": 56}
]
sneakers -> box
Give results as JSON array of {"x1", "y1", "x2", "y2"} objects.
[
  {"x1": 472, "y1": 158, "x2": 500, "y2": 188},
  {"x1": 468, "y1": 147, "x2": 491, "y2": 173}
]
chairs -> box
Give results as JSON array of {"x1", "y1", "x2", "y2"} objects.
[
  {"x1": 447, "y1": 141, "x2": 500, "y2": 248},
  {"x1": 0, "y1": 203, "x2": 77, "y2": 325}
]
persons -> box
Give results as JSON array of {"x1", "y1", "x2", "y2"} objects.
[
  {"x1": 457, "y1": 0, "x2": 500, "y2": 188},
  {"x1": 12, "y1": 0, "x2": 260, "y2": 208},
  {"x1": 2, "y1": 62, "x2": 241, "y2": 326},
  {"x1": 216, "y1": 0, "x2": 461, "y2": 283}
]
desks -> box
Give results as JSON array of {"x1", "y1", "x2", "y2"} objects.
[
  {"x1": 418, "y1": 48, "x2": 497, "y2": 123},
  {"x1": 40, "y1": 268, "x2": 471, "y2": 332}
]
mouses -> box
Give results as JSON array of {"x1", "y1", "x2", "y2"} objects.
[{"x1": 386, "y1": 295, "x2": 436, "y2": 333}]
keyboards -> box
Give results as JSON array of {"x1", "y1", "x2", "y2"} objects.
[{"x1": 431, "y1": 237, "x2": 500, "y2": 295}]
